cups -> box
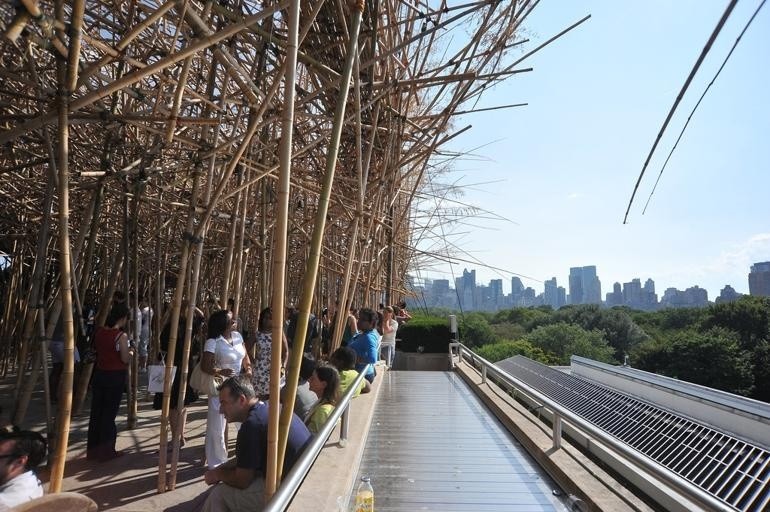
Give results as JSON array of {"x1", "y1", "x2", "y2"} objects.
[
  {"x1": 338, "y1": 493, "x2": 363, "y2": 511},
  {"x1": 563, "y1": 493, "x2": 589, "y2": 512}
]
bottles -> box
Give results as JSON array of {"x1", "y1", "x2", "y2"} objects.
[{"x1": 356, "y1": 476, "x2": 373, "y2": 512}]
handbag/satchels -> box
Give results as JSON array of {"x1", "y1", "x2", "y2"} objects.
[
  {"x1": 148, "y1": 365, "x2": 177, "y2": 392},
  {"x1": 190, "y1": 363, "x2": 223, "y2": 395}
]
